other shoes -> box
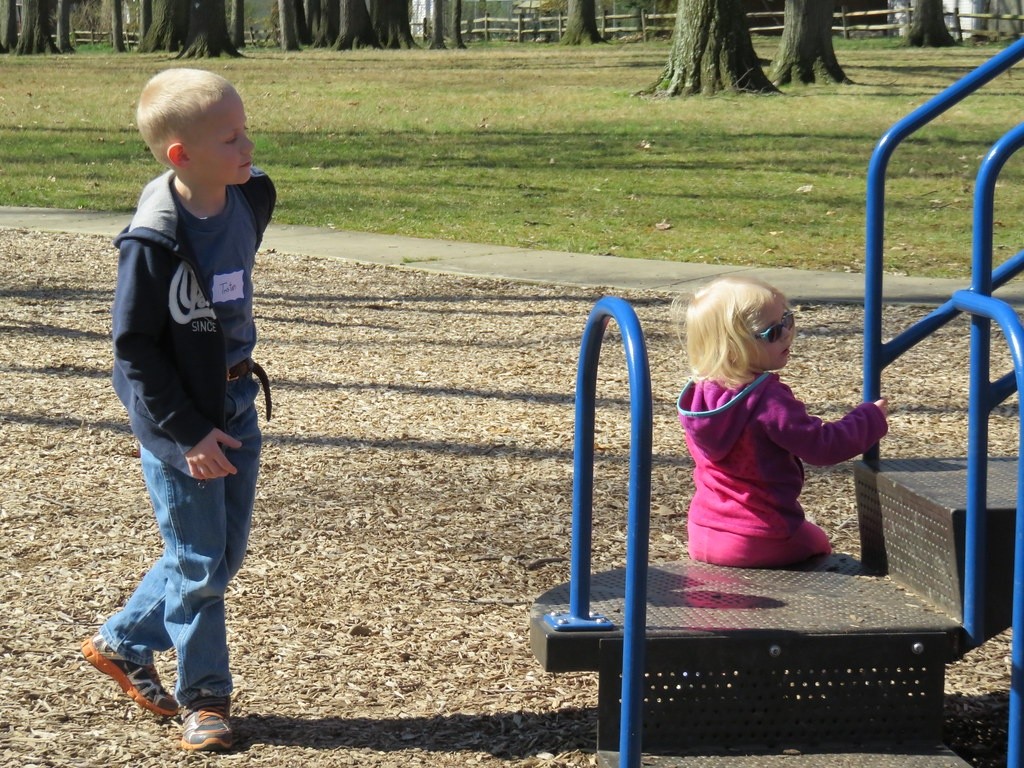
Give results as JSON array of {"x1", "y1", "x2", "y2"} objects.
[
  {"x1": 81, "y1": 632, "x2": 181, "y2": 716},
  {"x1": 181, "y1": 696, "x2": 235, "y2": 752}
]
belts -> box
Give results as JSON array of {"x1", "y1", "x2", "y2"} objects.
[{"x1": 227, "y1": 361, "x2": 273, "y2": 422}]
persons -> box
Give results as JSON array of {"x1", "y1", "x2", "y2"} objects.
[
  {"x1": 80, "y1": 68, "x2": 276, "y2": 750},
  {"x1": 671, "y1": 276, "x2": 889, "y2": 569}
]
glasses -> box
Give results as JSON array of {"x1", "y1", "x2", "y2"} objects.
[{"x1": 752, "y1": 311, "x2": 795, "y2": 343}]
